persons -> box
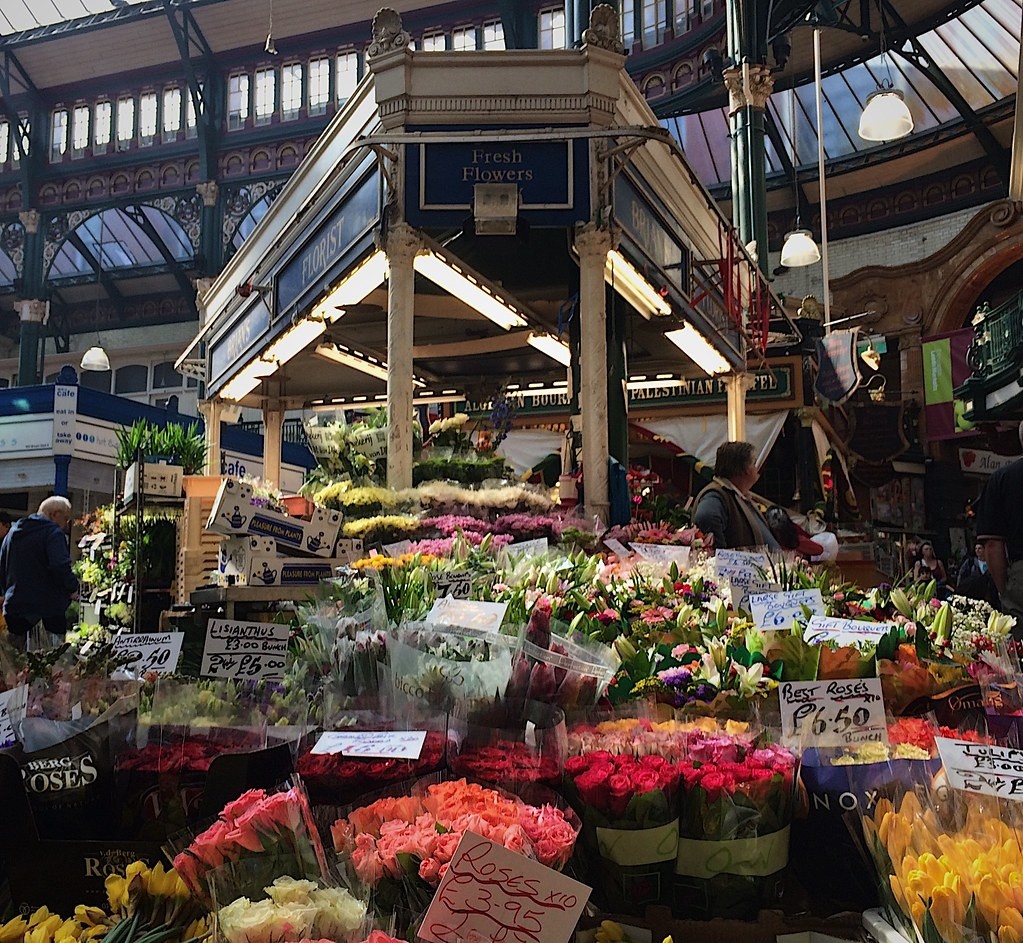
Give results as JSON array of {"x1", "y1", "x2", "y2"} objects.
[
  {"x1": 904, "y1": 535, "x2": 924, "y2": 584},
  {"x1": 767, "y1": 505, "x2": 823, "y2": 564},
  {"x1": 957, "y1": 542, "x2": 994, "y2": 598},
  {"x1": 0, "y1": 496, "x2": 81, "y2": 654},
  {"x1": 976, "y1": 421, "x2": 1023, "y2": 642},
  {"x1": 693, "y1": 442, "x2": 783, "y2": 555},
  {"x1": 914, "y1": 542, "x2": 946, "y2": 590},
  {"x1": 916, "y1": 566, "x2": 933, "y2": 594}
]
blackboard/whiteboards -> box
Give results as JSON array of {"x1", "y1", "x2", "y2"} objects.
[
  {"x1": 778, "y1": 678, "x2": 890, "y2": 750},
  {"x1": 714, "y1": 549, "x2": 769, "y2": 590},
  {"x1": 310, "y1": 730, "x2": 427, "y2": 761},
  {"x1": 200, "y1": 618, "x2": 290, "y2": 683},
  {"x1": 803, "y1": 614, "x2": 888, "y2": 660},
  {"x1": 507, "y1": 536, "x2": 549, "y2": 565},
  {"x1": 627, "y1": 541, "x2": 691, "y2": 572},
  {"x1": 748, "y1": 588, "x2": 827, "y2": 630},
  {"x1": 934, "y1": 736, "x2": 1023, "y2": 800},
  {"x1": 107, "y1": 631, "x2": 185, "y2": 681},
  {"x1": 603, "y1": 538, "x2": 630, "y2": 558},
  {"x1": 427, "y1": 570, "x2": 472, "y2": 598},
  {"x1": 418, "y1": 830, "x2": 592, "y2": 943}
]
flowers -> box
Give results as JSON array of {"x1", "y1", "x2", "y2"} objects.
[{"x1": 2, "y1": 377, "x2": 1022, "y2": 943}]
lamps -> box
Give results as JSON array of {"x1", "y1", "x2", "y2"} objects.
[
  {"x1": 857, "y1": 0, "x2": 915, "y2": 142},
  {"x1": 857, "y1": 373, "x2": 886, "y2": 403},
  {"x1": 80, "y1": 206, "x2": 110, "y2": 372},
  {"x1": 858, "y1": 331, "x2": 881, "y2": 371},
  {"x1": 778, "y1": 29, "x2": 821, "y2": 268}
]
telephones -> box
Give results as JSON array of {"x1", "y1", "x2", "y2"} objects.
[{"x1": 0, "y1": 684, "x2": 28, "y2": 746}]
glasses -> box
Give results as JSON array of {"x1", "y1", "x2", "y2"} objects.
[{"x1": 60, "y1": 513, "x2": 70, "y2": 524}]
